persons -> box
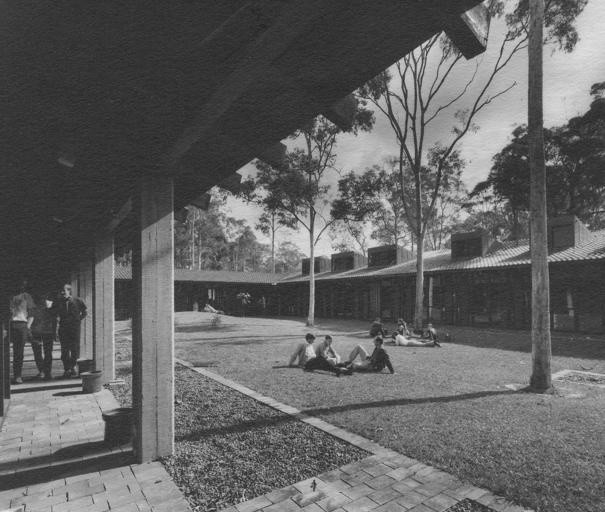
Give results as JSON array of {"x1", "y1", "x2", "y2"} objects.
[
  {"x1": 396, "y1": 318, "x2": 410, "y2": 335},
  {"x1": 368, "y1": 317, "x2": 386, "y2": 337},
  {"x1": 344, "y1": 335, "x2": 398, "y2": 375},
  {"x1": 315, "y1": 334, "x2": 340, "y2": 366},
  {"x1": 286, "y1": 333, "x2": 353, "y2": 377},
  {"x1": 26, "y1": 287, "x2": 55, "y2": 379},
  {"x1": 392, "y1": 331, "x2": 442, "y2": 348},
  {"x1": 8, "y1": 281, "x2": 34, "y2": 384},
  {"x1": 44, "y1": 283, "x2": 88, "y2": 377}
]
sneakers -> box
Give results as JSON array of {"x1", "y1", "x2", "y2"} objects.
[{"x1": 12, "y1": 366, "x2": 79, "y2": 385}]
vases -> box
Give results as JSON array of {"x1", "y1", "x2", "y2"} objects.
[
  {"x1": 78, "y1": 360, "x2": 94, "y2": 379},
  {"x1": 102, "y1": 407, "x2": 131, "y2": 448},
  {"x1": 79, "y1": 370, "x2": 104, "y2": 394}
]
